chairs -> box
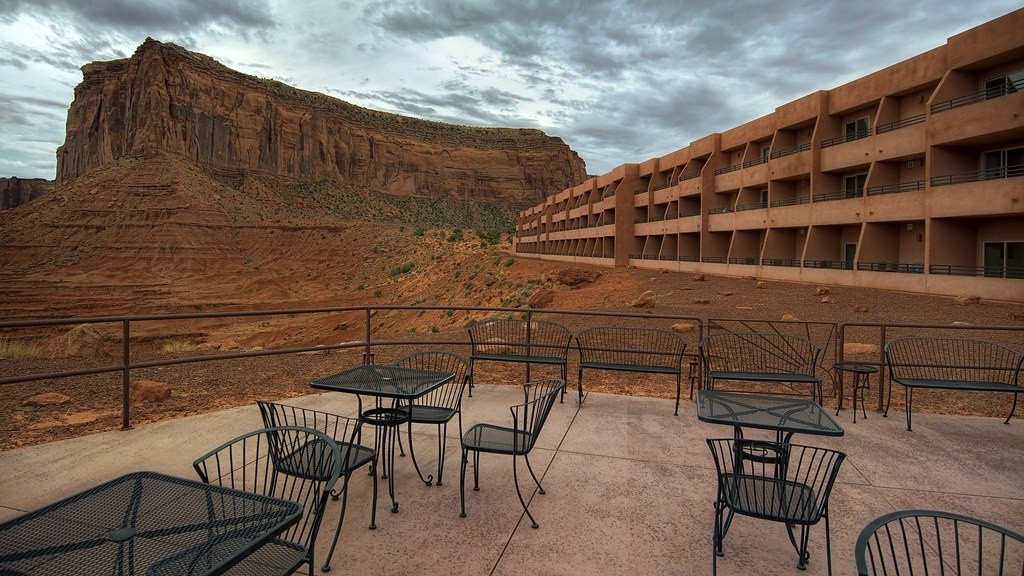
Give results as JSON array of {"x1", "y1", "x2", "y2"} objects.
[{"x1": 144, "y1": 350, "x2": 1024, "y2": 576}]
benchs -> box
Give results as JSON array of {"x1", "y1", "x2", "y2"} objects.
[
  {"x1": 467, "y1": 320, "x2": 572, "y2": 394},
  {"x1": 575, "y1": 326, "x2": 688, "y2": 416},
  {"x1": 883, "y1": 336, "x2": 1024, "y2": 431},
  {"x1": 698, "y1": 332, "x2": 823, "y2": 419}
]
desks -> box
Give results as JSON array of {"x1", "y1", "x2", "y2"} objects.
[
  {"x1": 832, "y1": 364, "x2": 880, "y2": 423},
  {"x1": 309, "y1": 364, "x2": 455, "y2": 513},
  {"x1": 0, "y1": 473, "x2": 303, "y2": 576},
  {"x1": 694, "y1": 391, "x2": 846, "y2": 527}
]
